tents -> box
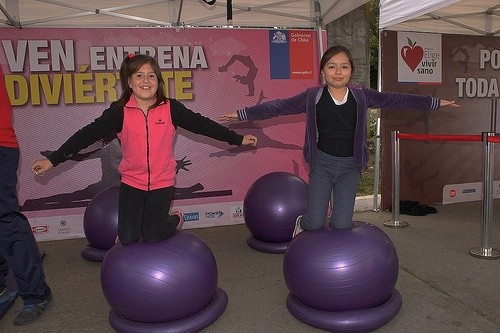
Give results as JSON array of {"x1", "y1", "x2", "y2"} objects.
[
  {"x1": 378, "y1": 0, "x2": 500, "y2": 213},
  {"x1": 0, "y1": 0, "x2": 372, "y2": 58}
]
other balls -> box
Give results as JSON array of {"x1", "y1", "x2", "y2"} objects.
[
  {"x1": 282, "y1": 220, "x2": 400, "y2": 310},
  {"x1": 83, "y1": 186, "x2": 118, "y2": 250},
  {"x1": 100, "y1": 230, "x2": 218, "y2": 321},
  {"x1": 243, "y1": 172, "x2": 309, "y2": 243}
]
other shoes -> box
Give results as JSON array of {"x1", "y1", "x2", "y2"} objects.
[
  {"x1": 0, "y1": 288, "x2": 6, "y2": 296},
  {"x1": 293, "y1": 215, "x2": 302, "y2": 238},
  {"x1": 172, "y1": 210, "x2": 184, "y2": 231},
  {"x1": 13, "y1": 294, "x2": 50, "y2": 325}
]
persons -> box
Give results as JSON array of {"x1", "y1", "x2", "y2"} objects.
[
  {"x1": 32, "y1": 53, "x2": 259, "y2": 246},
  {"x1": 1, "y1": 66, "x2": 53, "y2": 325},
  {"x1": 221, "y1": 46, "x2": 460, "y2": 236}
]
